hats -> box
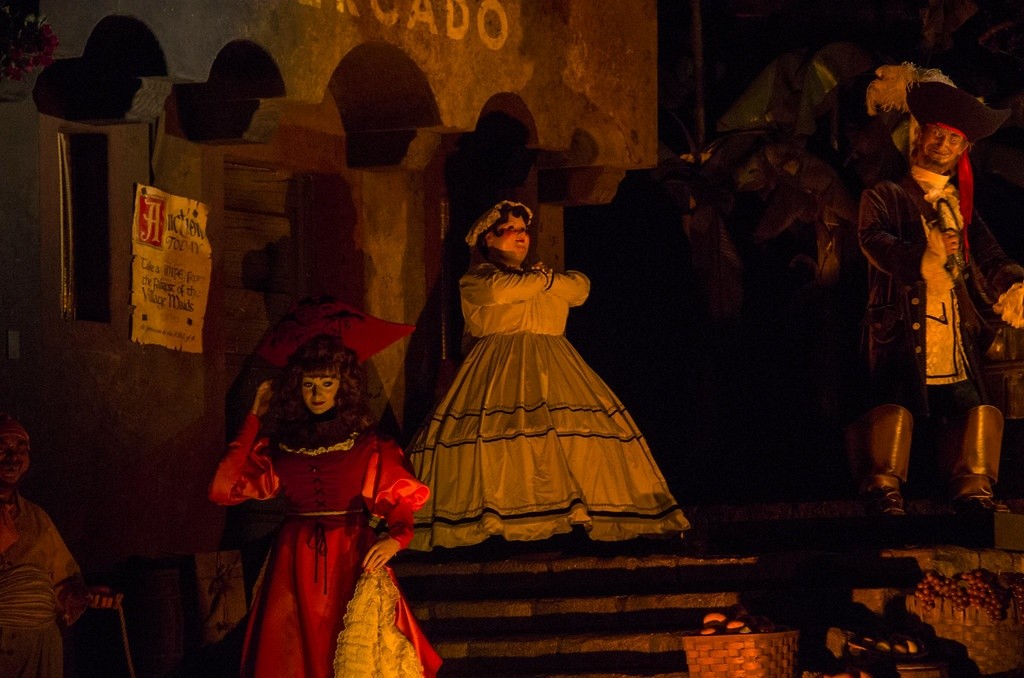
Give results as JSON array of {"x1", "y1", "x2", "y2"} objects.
[
  {"x1": 906, "y1": 81, "x2": 1013, "y2": 144},
  {"x1": 0, "y1": 415, "x2": 28, "y2": 440},
  {"x1": 465, "y1": 200, "x2": 533, "y2": 247},
  {"x1": 257, "y1": 294, "x2": 416, "y2": 379}
]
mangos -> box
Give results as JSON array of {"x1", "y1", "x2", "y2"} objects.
[{"x1": 874, "y1": 638, "x2": 920, "y2": 654}]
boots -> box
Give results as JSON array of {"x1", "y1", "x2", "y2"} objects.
[
  {"x1": 859, "y1": 404, "x2": 913, "y2": 516},
  {"x1": 949, "y1": 404, "x2": 1004, "y2": 510}
]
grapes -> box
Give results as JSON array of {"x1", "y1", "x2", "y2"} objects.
[{"x1": 915, "y1": 569, "x2": 1024, "y2": 629}]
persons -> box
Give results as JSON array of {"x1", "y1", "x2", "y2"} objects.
[
  {"x1": 206, "y1": 301, "x2": 443, "y2": 678},
  {"x1": 402, "y1": 200, "x2": 692, "y2": 553},
  {"x1": 856, "y1": 81, "x2": 1024, "y2": 516},
  {"x1": 0, "y1": 414, "x2": 121, "y2": 678}
]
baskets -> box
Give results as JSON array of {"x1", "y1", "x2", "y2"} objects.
[
  {"x1": 681, "y1": 627, "x2": 800, "y2": 678},
  {"x1": 905, "y1": 594, "x2": 1024, "y2": 678},
  {"x1": 826, "y1": 624, "x2": 929, "y2": 678}
]
